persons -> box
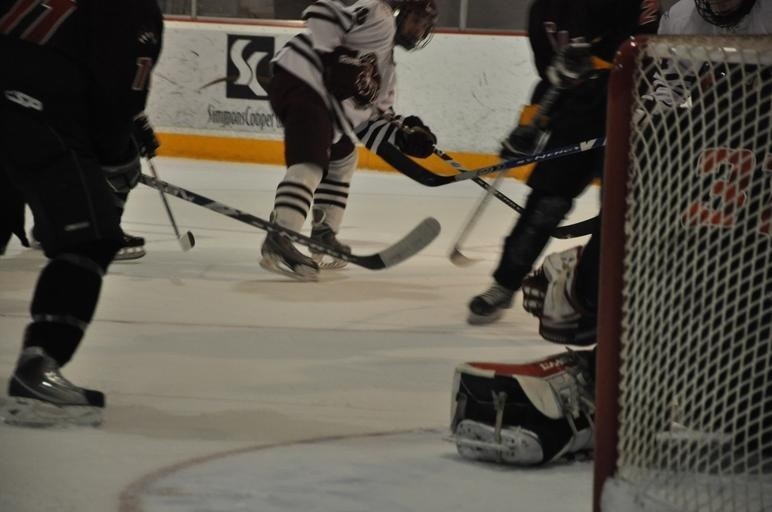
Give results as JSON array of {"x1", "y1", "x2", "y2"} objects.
[
  {"x1": 453, "y1": 61, "x2": 769, "y2": 471},
  {"x1": 634, "y1": 3, "x2": 769, "y2": 126},
  {"x1": 0, "y1": 0, "x2": 135, "y2": 408},
  {"x1": 465, "y1": 3, "x2": 663, "y2": 326},
  {"x1": 261, "y1": 1, "x2": 440, "y2": 266},
  {"x1": 30, "y1": 0, "x2": 165, "y2": 248}
]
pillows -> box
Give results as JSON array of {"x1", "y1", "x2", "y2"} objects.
[{"x1": 392, "y1": 0, "x2": 438, "y2": 50}]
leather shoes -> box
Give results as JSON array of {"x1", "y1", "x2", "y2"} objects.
[
  {"x1": 395, "y1": 115, "x2": 436, "y2": 158},
  {"x1": 323, "y1": 45, "x2": 354, "y2": 98}
]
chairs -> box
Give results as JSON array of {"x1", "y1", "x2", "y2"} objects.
[
  {"x1": 470, "y1": 282, "x2": 513, "y2": 316},
  {"x1": 10, "y1": 347, "x2": 104, "y2": 406},
  {"x1": 261, "y1": 208, "x2": 350, "y2": 272}
]
